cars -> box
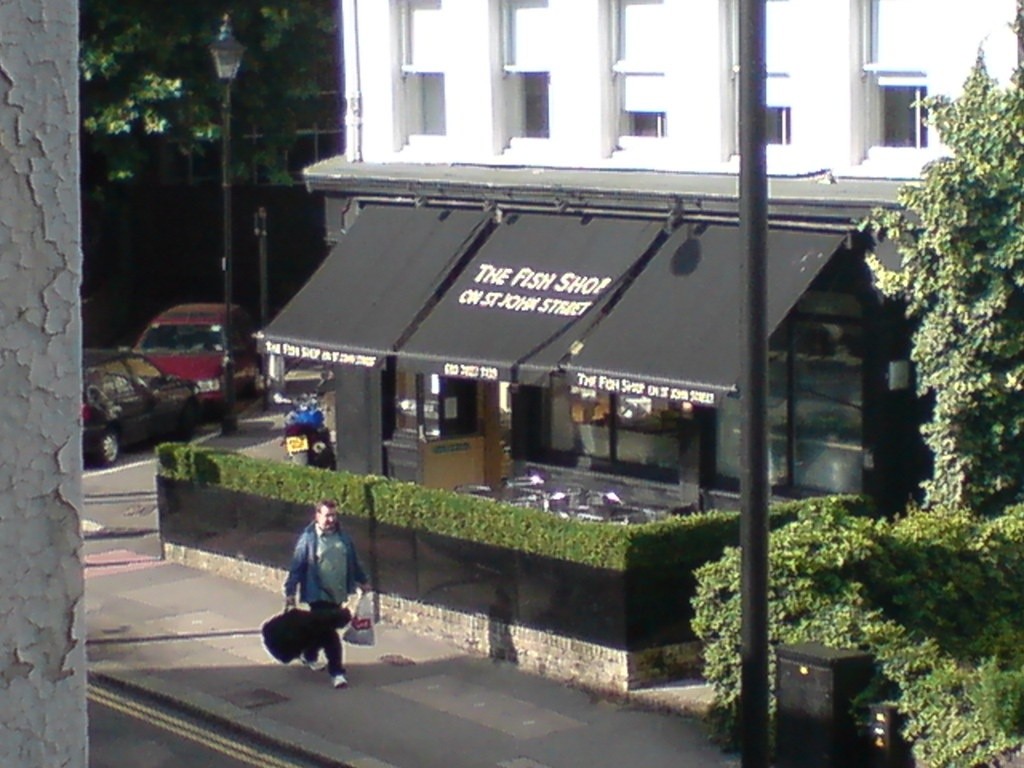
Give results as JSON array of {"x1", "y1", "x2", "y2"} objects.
[{"x1": 83, "y1": 347, "x2": 203, "y2": 468}]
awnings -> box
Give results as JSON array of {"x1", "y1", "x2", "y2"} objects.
[
  {"x1": 566, "y1": 220, "x2": 846, "y2": 409},
  {"x1": 256, "y1": 204, "x2": 494, "y2": 371},
  {"x1": 395, "y1": 211, "x2": 666, "y2": 391}
]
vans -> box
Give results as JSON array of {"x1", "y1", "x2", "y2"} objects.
[{"x1": 130, "y1": 303, "x2": 258, "y2": 419}]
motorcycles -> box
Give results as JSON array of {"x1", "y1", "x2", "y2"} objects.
[{"x1": 274, "y1": 371, "x2": 338, "y2": 473}]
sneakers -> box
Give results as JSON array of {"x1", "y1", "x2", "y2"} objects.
[
  {"x1": 301, "y1": 651, "x2": 324, "y2": 671},
  {"x1": 332, "y1": 673, "x2": 348, "y2": 688}
]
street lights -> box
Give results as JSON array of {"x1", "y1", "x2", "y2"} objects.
[{"x1": 209, "y1": 11, "x2": 244, "y2": 438}]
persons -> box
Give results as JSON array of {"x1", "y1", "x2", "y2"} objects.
[{"x1": 284, "y1": 500, "x2": 370, "y2": 689}]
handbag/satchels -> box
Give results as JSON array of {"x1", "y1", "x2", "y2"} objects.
[
  {"x1": 261, "y1": 601, "x2": 315, "y2": 663},
  {"x1": 342, "y1": 588, "x2": 375, "y2": 645}
]
